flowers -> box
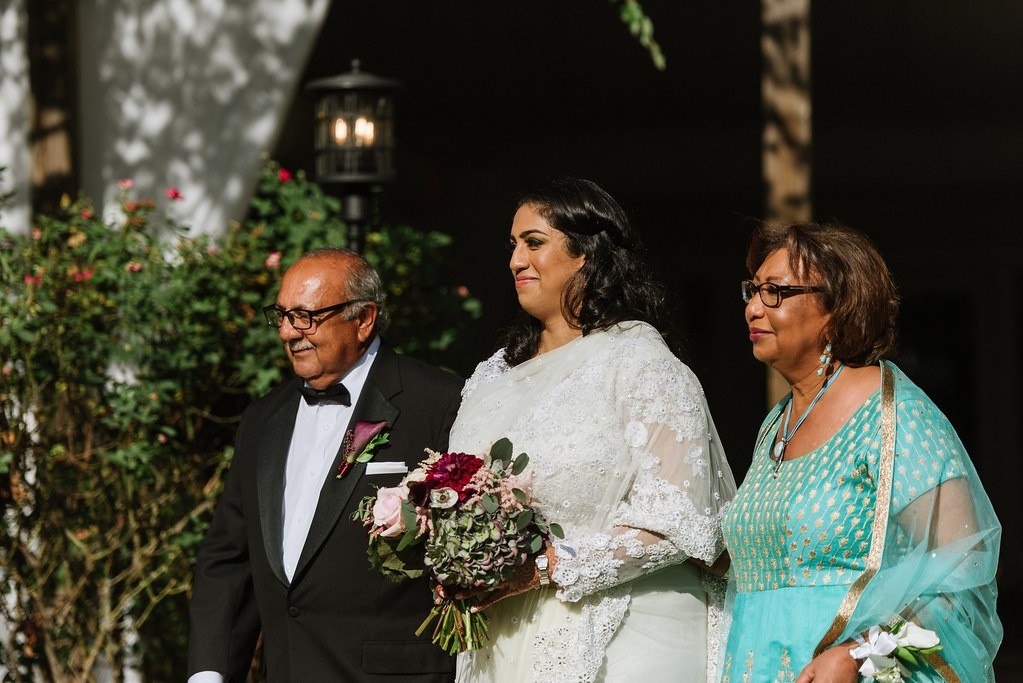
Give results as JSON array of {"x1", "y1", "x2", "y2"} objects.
[
  {"x1": 351, "y1": 438, "x2": 568, "y2": 658},
  {"x1": 848, "y1": 615, "x2": 945, "y2": 683}
]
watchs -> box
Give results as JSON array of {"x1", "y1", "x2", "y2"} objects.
[{"x1": 535, "y1": 548, "x2": 551, "y2": 585}]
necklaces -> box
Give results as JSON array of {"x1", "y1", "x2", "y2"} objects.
[{"x1": 773, "y1": 363, "x2": 845, "y2": 478}]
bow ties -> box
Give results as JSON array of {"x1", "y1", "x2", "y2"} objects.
[{"x1": 295, "y1": 384, "x2": 350, "y2": 406}]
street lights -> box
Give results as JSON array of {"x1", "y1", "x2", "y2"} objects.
[{"x1": 309, "y1": 57, "x2": 397, "y2": 257}]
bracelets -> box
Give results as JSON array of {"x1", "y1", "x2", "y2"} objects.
[{"x1": 850, "y1": 632, "x2": 869, "y2": 665}]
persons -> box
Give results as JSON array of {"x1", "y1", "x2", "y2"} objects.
[
  {"x1": 445, "y1": 177, "x2": 737, "y2": 683},
  {"x1": 719, "y1": 220, "x2": 1005, "y2": 683},
  {"x1": 185, "y1": 250, "x2": 466, "y2": 682}
]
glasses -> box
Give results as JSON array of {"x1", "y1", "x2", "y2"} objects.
[
  {"x1": 262, "y1": 297, "x2": 361, "y2": 330},
  {"x1": 741, "y1": 278, "x2": 824, "y2": 308}
]
